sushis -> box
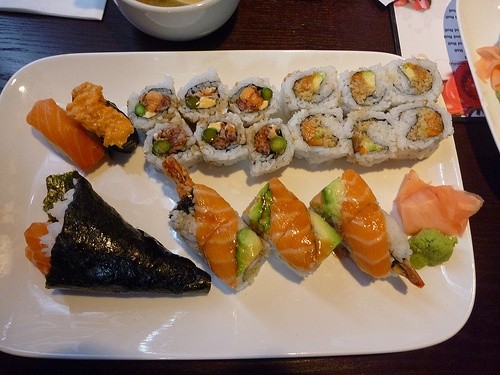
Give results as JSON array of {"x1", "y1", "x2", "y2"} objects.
[
  {"x1": 192, "y1": 110, "x2": 249, "y2": 165},
  {"x1": 281, "y1": 63, "x2": 340, "y2": 111},
  {"x1": 385, "y1": 102, "x2": 456, "y2": 160},
  {"x1": 242, "y1": 176, "x2": 345, "y2": 280},
  {"x1": 143, "y1": 114, "x2": 203, "y2": 173},
  {"x1": 344, "y1": 108, "x2": 396, "y2": 167},
  {"x1": 63, "y1": 79, "x2": 138, "y2": 152},
  {"x1": 244, "y1": 117, "x2": 295, "y2": 176},
  {"x1": 287, "y1": 107, "x2": 348, "y2": 164},
  {"x1": 126, "y1": 74, "x2": 180, "y2": 129},
  {"x1": 177, "y1": 68, "x2": 228, "y2": 121},
  {"x1": 24, "y1": 169, "x2": 213, "y2": 293},
  {"x1": 227, "y1": 75, "x2": 279, "y2": 125},
  {"x1": 161, "y1": 157, "x2": 273, "y2": 291},
  {"x1": 309, "y1": 170, "x2": 426, "y2": 292},
  {"x1": 382, "y1": 55, "x2": 443, "y2": 106},
  {"x1": 338, "y1": 63, "x2": 392, "y2": 113}
]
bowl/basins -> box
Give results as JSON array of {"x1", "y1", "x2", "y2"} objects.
[{"x1": 114, "y1": 1, "x2": 238, "y2": 40}]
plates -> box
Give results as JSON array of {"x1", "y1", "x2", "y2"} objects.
[
  {"x1": 457, "y1": 1, "x2": 500, "y2": 162},
  {"x1": 0, "y1": 49, "x2": 477, "y2": 361}
]
